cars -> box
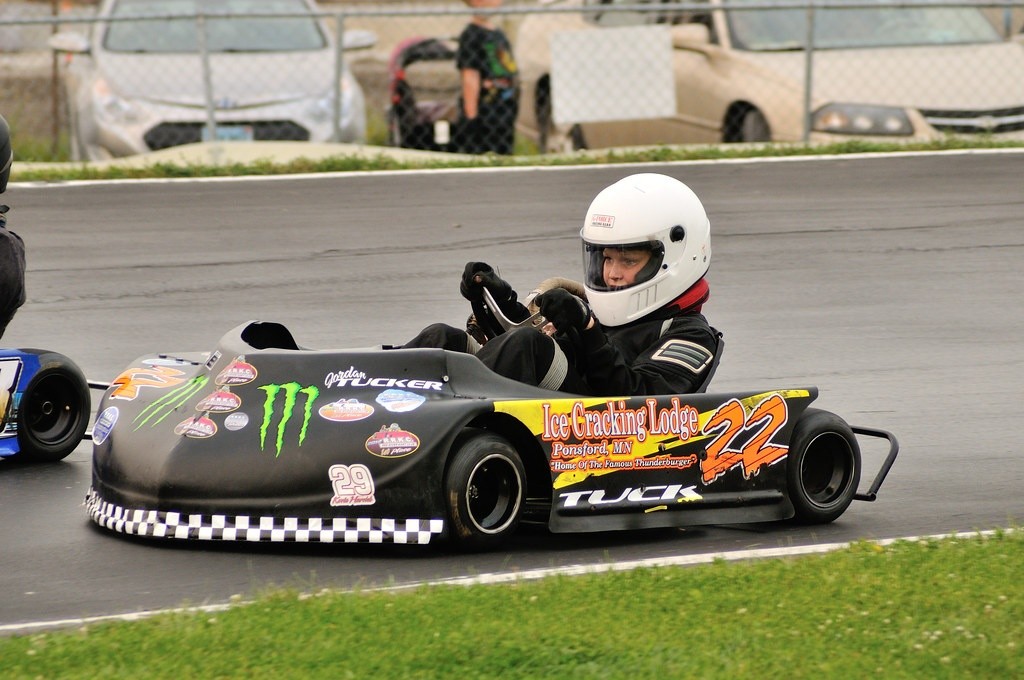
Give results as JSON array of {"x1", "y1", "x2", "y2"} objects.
[
  {"x1": 512, "y1": 0, "x2": 1024, "y2": 156},
  {"x1": 47, "y1": 1, "x2": 378, "y2": 169}
]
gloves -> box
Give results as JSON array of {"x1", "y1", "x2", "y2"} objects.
[
  {"x1": 459, "y1": 261, "x2": 512, "y2": 303},
  {"x1": 535, "y1": 288, "x2": 592, "y2": 334}
]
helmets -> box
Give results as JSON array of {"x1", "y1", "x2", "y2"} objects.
[
  {"x1": 0, "y1": 114, "x2": 13, "y2": 194},
  {"x1": 578, "y1": 173, "x2": 712, "y2": 329}
]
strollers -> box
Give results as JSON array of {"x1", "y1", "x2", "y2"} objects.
[{"x1": 386, "y1": 35, "x2": 463, "y2": 152}]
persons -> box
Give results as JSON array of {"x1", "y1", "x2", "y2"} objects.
[
  {"x1": 0, "y1": 115, "x2": 26, "y2": 340},
  {"x1": 400, "y1": 173, "x2": 717, "y2": 396},
  {"x1": 456, "y1": 0, "x2": 520, "y2": 154}
]
